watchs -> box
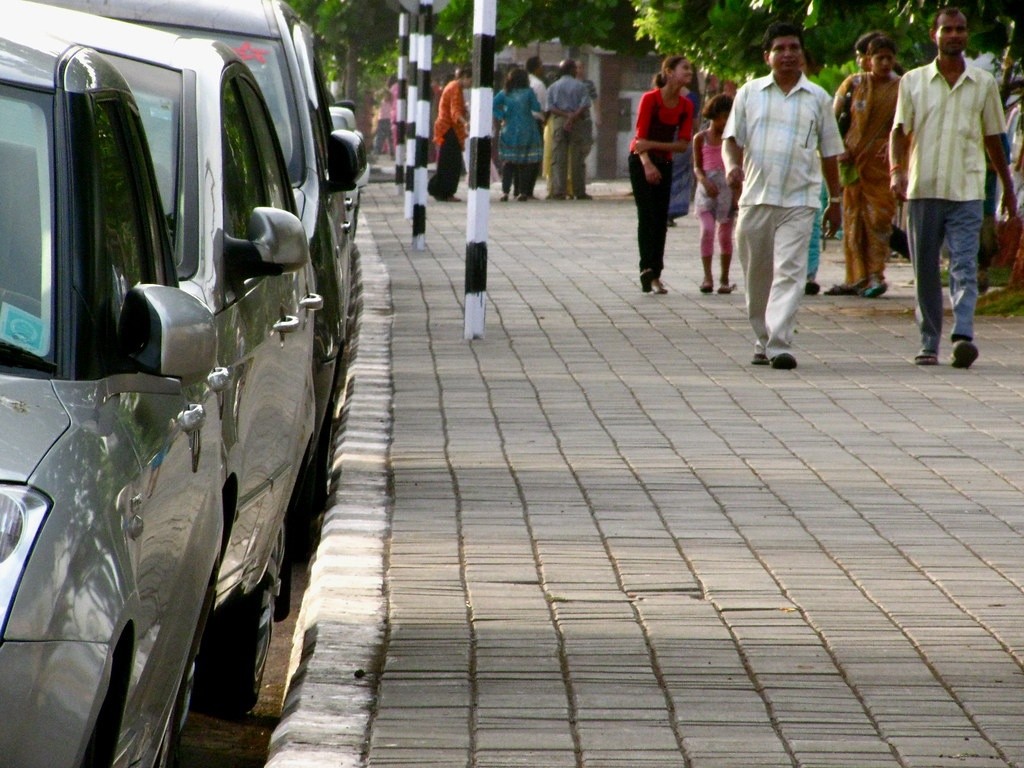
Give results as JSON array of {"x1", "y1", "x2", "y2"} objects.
[{"x1": 826, "y1": 196, "x2": 843, "y2": 204}]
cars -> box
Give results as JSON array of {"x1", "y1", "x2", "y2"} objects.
[{"x1": 1, "y1": 0, "x2": 371, "y2": 768}]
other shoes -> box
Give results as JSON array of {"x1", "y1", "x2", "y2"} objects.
[
  {"x1": 750, "y1": 354, "x2": 769, "y2": 365},
  {"x1": 501, "y1": 193, "x2": 529, "y2": 201},
  {"x1": 977, "y1": 263, "x2": 989, "y2": 293},
  {"x1": 640, "y1": 268, "x2": 653, "y2": 292},
  {"x1": 772, "y1": 353, "x2": 796, "y2": 369},
  {"x1": 718, "y1": 278, "x2": 730, "y2": 294},
  {"x1": 447, "y1": 195, "x2": 460, "y2": 202},
  {"x1": 651, "y1": 282, "x2": 667, "y2": 294},
  {"x1": 700, "y1": 276, "x2": 713, "y2": 293},
  {"x1": 805, "y1": 281, "x2": 820, "y2": 295},
  {"x1": 667, "y1": 216, "x2": 673, "y2": 226}
]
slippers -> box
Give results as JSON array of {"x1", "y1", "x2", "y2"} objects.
[
  {"x1": 951, "y1": 340, "x2": 978, "y2": 369},
  {"x1": 914, "y1": 347, "x2": 938, "y2": 365},
  {"x1": 855, "y1": 283, "x2": 888, "y2": 298},
  {"x1": 823, "y1": 283, "x2": 863, "y2": 295}
]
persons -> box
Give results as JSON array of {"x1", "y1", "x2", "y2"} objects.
[
  {"x1": 665, "y1": 16, "x2": 1016, "y2": 296},
  {"x1": 721, "y1": 23, "x2": 843, "y2": 370},
  {"x1": 887, "y1": 7, "x2": 1016, "y2": 367},
  {"x1": 376, "y1": 57, "x2": 594, "y2": 202},
  {"x1": 629, "y1": 56, "x2": 693, "y2": 294}
]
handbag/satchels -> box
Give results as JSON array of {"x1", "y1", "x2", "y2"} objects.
[{"x1": 836, "y1": 83, "x2": 854, "y2": 140}]
